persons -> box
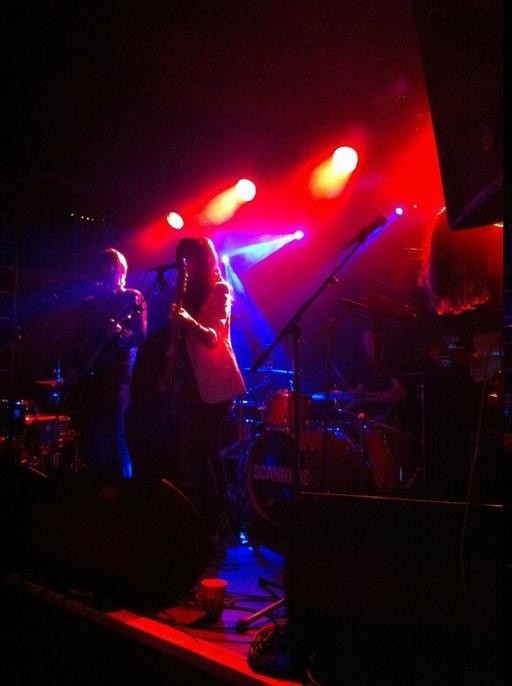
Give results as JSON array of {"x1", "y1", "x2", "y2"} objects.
[
  {"x1": 343, "y1": 328, "x2": 407, "y2": 413},
  {"x1": 156, "y1": 237, "x2": 248, "y2": 552},
  {"x1": 65, "y1": 248, "x2": 148, "y2": 486}
]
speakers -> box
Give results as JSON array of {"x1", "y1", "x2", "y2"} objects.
[
  {"x1": 0, "y1": 471, "x2": 214, "y2": 615},
  {"x1": 415, "y1": 0, "x2": 511, "y2": 230}
]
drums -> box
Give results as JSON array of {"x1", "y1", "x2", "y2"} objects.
[
  {"x1": 366, "y1": 415, "x2": 414, "y2": 493},
  {"x1": 243, "y1": 422, "x2": 368, "y2": 528},
  {"x1": 266, "y1": 389, "x2": 313, "y2": 430}
]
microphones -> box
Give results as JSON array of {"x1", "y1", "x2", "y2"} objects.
[
  {"x1": 350, "y1": 215, "x2": 388, "y2": 243},
  {"x1": 153, "y1": 262, "x2": 178, "y2": 271}
]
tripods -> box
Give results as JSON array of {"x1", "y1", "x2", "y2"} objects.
[{"x1": 236, "y1": 242, "x2": 366, "y2": 633}]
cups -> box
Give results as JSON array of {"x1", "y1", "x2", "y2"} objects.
[{"x1": 201, "y1": 578, "x2": 229, "y2": 612}]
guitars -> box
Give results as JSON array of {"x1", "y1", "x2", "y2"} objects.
[{"x1": 156, "y1": 266, "x2": 188, "y2": 398}]
